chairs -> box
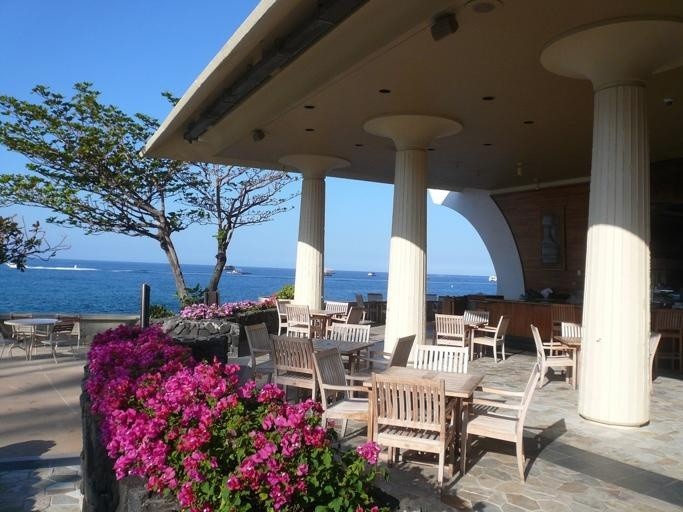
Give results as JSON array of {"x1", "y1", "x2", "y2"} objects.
[
  {"x1": 459, "y1": 362, "x2": 540, "y2": 485},
  {"x1": 0, "y1": 312, "x2": 81, "y2": 365},
  {"x1": 244, "y1": 294, "x2": 510, "y2": 402},
  {"x1": 649, "y1": 333, "x2": 661, "y2": 390},
  {"x1": 561, "y1": 322, "x2": 582, "y2": 337},
  {"x1": 312, "y1": 345, "x2": 392, "y2": 472},
  {"x1": 531, "y1": 324, "x2": 577, "y2": 390},
  {"x1": 371, "y1": 372, "x2": 458, "y2": 499},
  {"x1": 651, "y1": 310, "x2": 683, "y2": 374},
  {"x1": 550, "y1": 304, "x2": 575, "y2": 356}
]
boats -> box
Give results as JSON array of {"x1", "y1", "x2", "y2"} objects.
[
  {"x1": 5, "y1": 262, "x2": 27, "y2": 268},
  {"x1": 324, "y1": 268, "x2": 336, "y2": 277},
  {"x1": 489, "y1": 275, "x2": 497, "y2": 283},
  {"x1": 367, "y1": 271, "x2": 376, "y2": 276},
  {"x1": 223, "y1": 265, "x2": 251, "y2": 274}
]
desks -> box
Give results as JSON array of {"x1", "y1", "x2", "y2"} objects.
[
  {"x1": 554, "y1": 336, "x2": 582, "y2": 346},
  {"x1": 362, "y1": 366, "x2": 485, "y2": 477}
]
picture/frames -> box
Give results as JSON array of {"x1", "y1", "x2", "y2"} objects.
[{"x1": 537, "y1": 206, "x2": 565, "y2": 271}]
